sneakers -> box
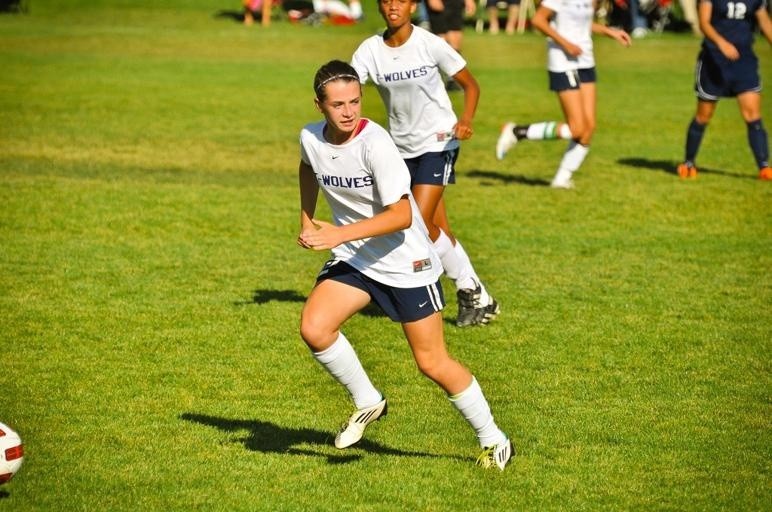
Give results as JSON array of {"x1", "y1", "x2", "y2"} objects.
[
  {"x1": 475, "y1": 439, "x2": 514, "y2": 470},
  {"x1": 333, "y1": 395, "x2": 388, "y2": 449},
  {"x1": 551, "y1": 179, "x2": 574, "y2": 189},
  {"x1": 453, "y1": 278, "x2": 482, "y2": 329},
  {"x1": 476, "y1": 295, "x2": 500, "y2": 324},
  {"x1": 759, "y1": 166, "x2": 771, "y2": 179},
  {"x1": 677, "y1": 162, "x2": 697, "y2": 178},
  {"x1": 494, "y1": 120, "x2": 518, "y2": 161}
]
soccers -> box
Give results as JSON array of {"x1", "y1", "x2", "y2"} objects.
[{"x1": 0, "y1": 423, "x2": 24, "y2": 486}]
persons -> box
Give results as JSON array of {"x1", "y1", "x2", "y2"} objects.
[
  {"x1": 494, "y1": 1, "x2": 632, "y2": 189},
  {"x1": 675, "y1": 1, "x2": 772, "y2": 182},
  {"x1": 350, "y1": 1, "x2": 503, "y2": 327},
  {"x1": 296, "y1": 58, "x2": 516, "y2": 471}
]
